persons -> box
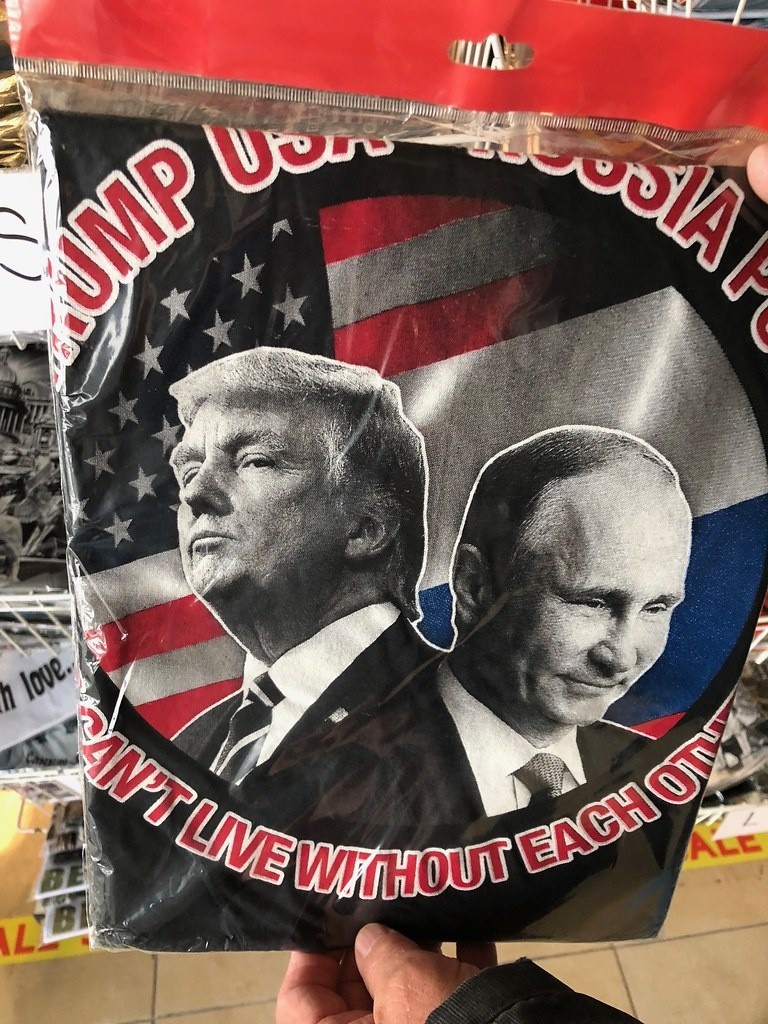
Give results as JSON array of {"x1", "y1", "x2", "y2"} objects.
[
  {"x1": 167, "y1": 348, "x2": 692, "y2": 831},
  {"x1": 275, "y1": 922, "x2": 643, "y2": 1024}
]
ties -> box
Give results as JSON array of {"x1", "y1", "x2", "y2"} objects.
[
  {"x1": 208, "y1": 674, "x2": 285, "y2": 780},
  {"x1": 511, "y1": 752, "x2": 567, "y2": 804}
]
